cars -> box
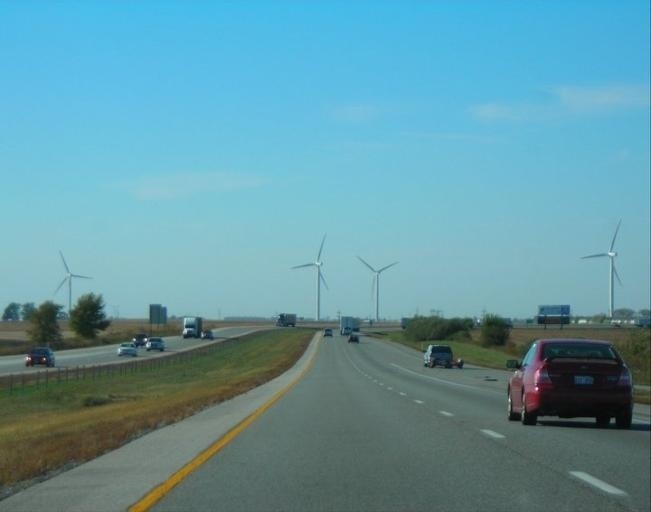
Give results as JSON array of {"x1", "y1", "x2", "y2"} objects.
[
  {"x1": 117, "y1": 333, "x2": 165, "y2": 357},
  {"x1": 505, "y1": 338, "x2": 634, "y2": 428},
  {"x1": 347, "y1": 334, "x2": 360, "y2": 344},
  {"x1": 323, "y1": 328, "x2": 333, "y2": 337},
  {"x1": 200, "y1": 328, "x2": 214, "y2": 340},
  {"x1": 423, "y1": 343, "x2": 453, "y2": 368},
  {"x1": 25, "y1": 347, "x2": 55, "y2": 367}
]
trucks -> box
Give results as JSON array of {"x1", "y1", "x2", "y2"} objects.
[
  {"x1": 182, "y1": 316, "x2": 202, "y2": 338},
  {"x1": 339, "y1": 316, "x2": 361, "y2": 335},
  {"x1": 272, "y1": 312, "x2": 296, "y2": 327}
]
